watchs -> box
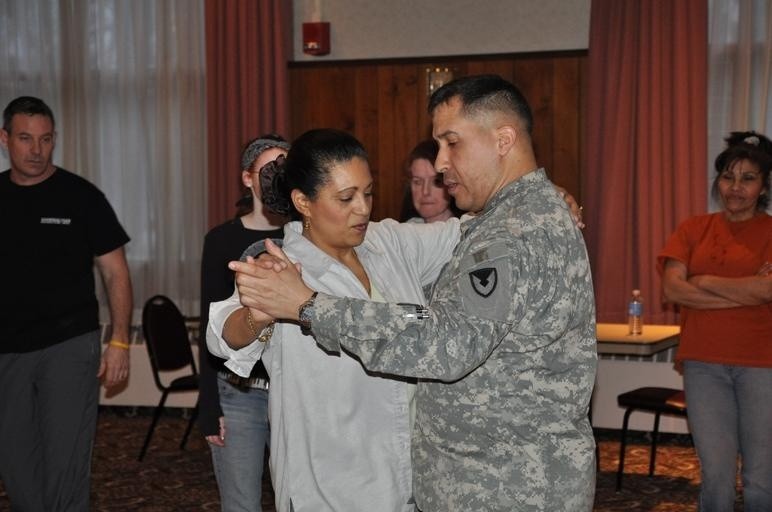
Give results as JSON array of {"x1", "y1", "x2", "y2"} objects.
[{"x1": 296, "y1": 291, "x2": 321, "y2": 337}]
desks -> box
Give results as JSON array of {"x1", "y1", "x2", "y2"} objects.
[{"x1": 596, "y1": 324, "x2": 681, "y2": 362}]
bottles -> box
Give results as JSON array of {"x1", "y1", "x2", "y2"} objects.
[{"x1": 628, "y1": 289, "x2": 644, "y2": 335}]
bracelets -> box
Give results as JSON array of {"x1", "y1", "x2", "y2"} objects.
[{"x1": 245, "y1": 307, "x2": 276, "y2": 343}]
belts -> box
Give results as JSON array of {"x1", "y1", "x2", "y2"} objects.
[{"x1": 215, "y1": 369, "x2": 269, "y2": 391}]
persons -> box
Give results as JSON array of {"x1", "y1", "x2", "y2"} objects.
[
  {"x1": 199, "y1": 134, "x2": 301, "y2": 511},
  {"x1": 654, "y1": 130, "x2": 772, "y2": 511},
  {"x1": 398, "y1": 139, "x2": 470, "y2": 224},
  {"x1": 0, "y1": 96, "x2": 133, "y2": 512},
  {"x1": 205, "y1": 127, "x2": 588, "y2": 512},
  {"x1": 227, "y1": 72, "x2": 598, "y2": 512}
]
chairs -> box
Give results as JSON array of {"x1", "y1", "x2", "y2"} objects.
[
  {"x1": 139, "y1": 294, "x2": 202, "y2": 463},
  {"x1": 617, "y1": 386, "x2": 689, "y2": 494}
]
